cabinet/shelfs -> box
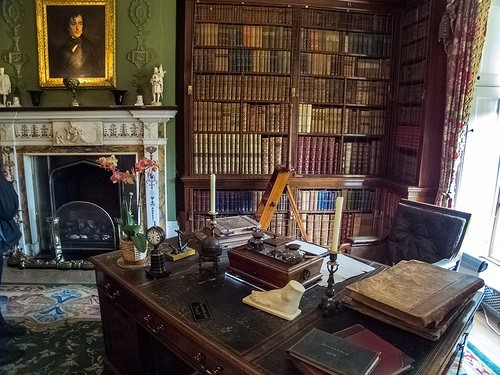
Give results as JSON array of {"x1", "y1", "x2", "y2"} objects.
[{"x1": 175, "y1": 0, "x2": 443, "y2": 238}]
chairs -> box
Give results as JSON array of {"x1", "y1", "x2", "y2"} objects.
[{"x1": 338, "y1": 198, "x2": 472, "y2": 271}]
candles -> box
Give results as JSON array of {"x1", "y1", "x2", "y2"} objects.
[{"x1": 210, "y1": 174, "x2": 216, "y2": 212}]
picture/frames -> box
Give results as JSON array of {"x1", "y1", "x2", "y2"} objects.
[{"x1": 36, "y1": 0, "x2": 117, "y2": 88}]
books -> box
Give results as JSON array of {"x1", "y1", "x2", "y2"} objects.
[
  {"x1": 190, "y1": 0, "x2": 433, "y2": 246},
  {"x1": 287, "y1": 324, "x2": 414, "y2": 375},
  {"x1": 344, "y1": 259, "x2": 484, "y2": 341}
]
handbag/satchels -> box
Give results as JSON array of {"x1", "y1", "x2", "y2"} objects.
[
  {"x1": 0, "y1": 217, "x2": 22, "y2": 252},
  {"x1": 0, "y1": 171, "x2": 19, "y2": 221}
]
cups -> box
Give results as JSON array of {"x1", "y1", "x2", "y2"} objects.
[
  {"x1": 27, "y1": 90, "x2": 44, "y2": 106},
  {"x1": 111, "y1": 89, "x2": 128, "y2": 105}
]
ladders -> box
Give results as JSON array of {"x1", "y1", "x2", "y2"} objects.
[{"x1": 255, "y1": 163, "x2": 309, "y2": 243}]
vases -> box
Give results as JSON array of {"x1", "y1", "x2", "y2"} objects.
[{"x1": 119, "y1": 238, "x2": 149, "y2": 262}]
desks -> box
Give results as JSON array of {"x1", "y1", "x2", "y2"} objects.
[{"x1": 87, "y1": 229, "x2": 486, "y2": 375}]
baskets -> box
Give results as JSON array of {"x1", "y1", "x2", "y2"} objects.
[{"x1": 121, "y1": 239, "x2": 149, "y2": 261}]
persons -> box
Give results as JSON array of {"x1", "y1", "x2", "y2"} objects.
[{"x1": 0, "y1": 171, "x2": 26, "y2": 366}]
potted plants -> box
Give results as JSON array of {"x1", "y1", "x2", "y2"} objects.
[{"x1": 331, "y1": 196, "x2": 343, "y2": 252}]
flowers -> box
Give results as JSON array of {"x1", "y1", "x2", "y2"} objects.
[{"x1": 96, "y1": 155, "x2": 154, "y2": 253}]
